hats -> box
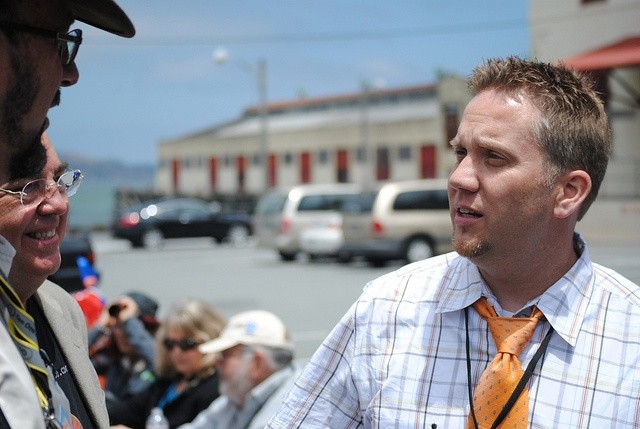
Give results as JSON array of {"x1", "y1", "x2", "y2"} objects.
[
  {"x1": 24, "y1": 0, "x2": 134, "y2": 37},
  {"x1": 195, "y1": 309, "x2": 292, "y2": 356},
  {"x1": 119, "y1": 288, "x2": 163, "y2": 332}
]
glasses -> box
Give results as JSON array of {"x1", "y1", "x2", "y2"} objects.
[
  {"x1": 0, "y1": 16, "x2": 83, "y2": 69},
  {"x1": 160, "y1": 336, "x2": 202, "y2": 352},
  {"x1": 1, "y1": 168, "x2": 86, "y2": 209}
]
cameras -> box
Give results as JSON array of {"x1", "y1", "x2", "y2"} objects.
[{"x1": 106, "y1": 302, "x2": 124, "y2": 317}]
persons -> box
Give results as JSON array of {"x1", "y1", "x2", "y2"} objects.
[
  {"x1": 87, "y1": 290, "x2": 175, "y2": 429},
  {"x1": 263, "y1": 59, "x2": 638, "y2": 429},
  {"x1": 0, "y1": 127, "x2": 110, "y2": 429},
  {"x1": 110, "y1": 296, "x2": 229, "y2": 429},
  {"x1": 177, "y1": 311, "x2": 301, "y2": 429},
  {"x1": 0, "y1": 1, "x2": 137, "y2": 189}
]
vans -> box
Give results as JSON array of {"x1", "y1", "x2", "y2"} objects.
[
  {"x1": 263, "y1": 183, "x2": 363, "y2": 264},
  {"x1": 342, "y1": 179, "x2": 454, "y2": 263}
]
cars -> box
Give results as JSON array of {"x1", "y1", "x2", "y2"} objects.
[
  {"x1": 47, "y1": 234, "x2": 95, "y2": 293},
  {"x1": 113, "y1": 195, "x2": 254, "y2": 248}
]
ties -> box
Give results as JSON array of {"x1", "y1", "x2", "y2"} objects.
[{"x1": 464, "y1": 298, "x2": 544, "y2": 429}]
